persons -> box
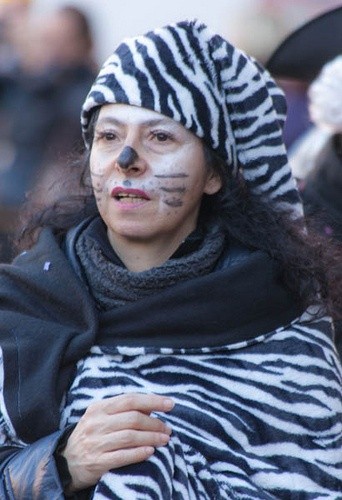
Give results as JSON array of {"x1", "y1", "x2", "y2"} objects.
[
  {"x1": 0, "y1": 0, "x2": 100, "y2": 265},
  {"x1": 0, "y1": 17, "x2": 342, "y2": 500}
]
